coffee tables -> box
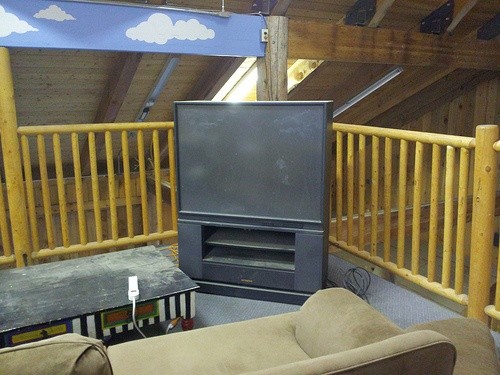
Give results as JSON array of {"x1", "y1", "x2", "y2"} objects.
[{"x1": 0, "y1": 244, "x2": 201, "y2": 348}]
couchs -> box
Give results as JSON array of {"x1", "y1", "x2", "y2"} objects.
[{"x1": 0, "y1": 287, "x2": 458, "y2": 375}]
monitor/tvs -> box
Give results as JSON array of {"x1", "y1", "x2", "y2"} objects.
[{"x1": 173, "y1": 99, "x2": 333, "y2": 230}]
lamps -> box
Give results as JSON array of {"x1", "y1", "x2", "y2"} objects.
[
  {"x1": 330, "y1": 65, "x2": 405, "y2": 120},
  {"x1": 125, "y1": 54, "x2": 178, "y2": 137}
]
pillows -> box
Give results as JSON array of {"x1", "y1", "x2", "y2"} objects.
[{"x1": 405, "y1": 318, "x2": 500, "y2": 375}]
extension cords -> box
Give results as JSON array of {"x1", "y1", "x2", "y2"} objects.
[{"x1": 127, "y1": 275, "x2": 139, "y2": 301}]
